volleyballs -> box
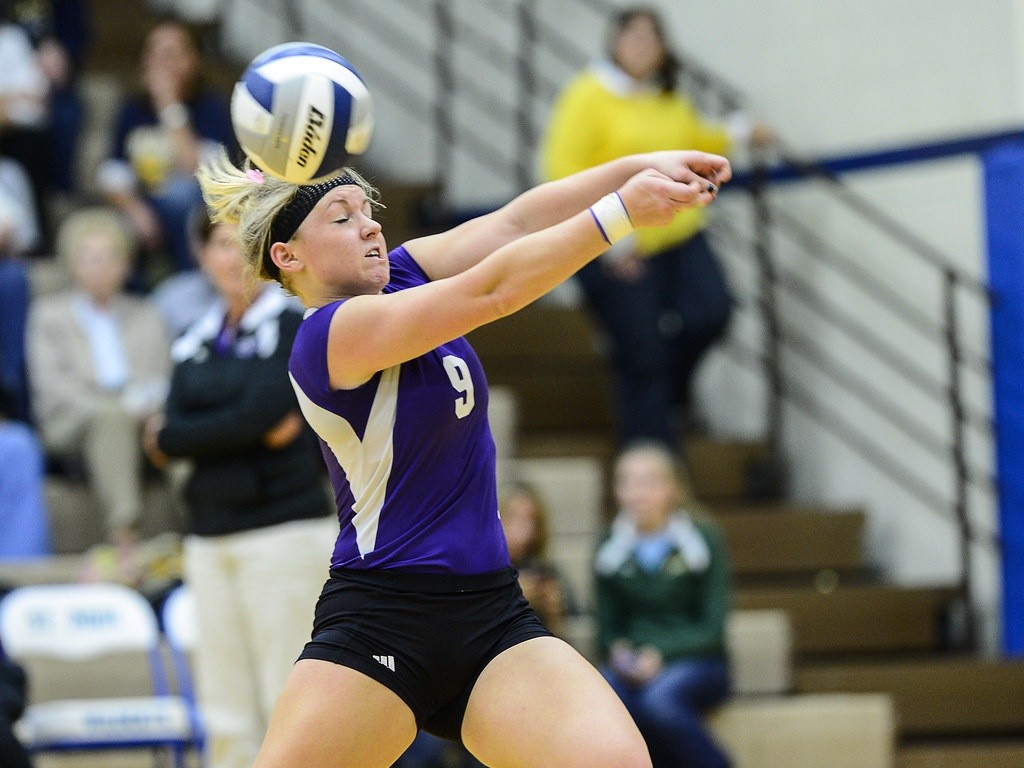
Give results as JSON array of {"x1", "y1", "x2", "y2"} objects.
[{"x1": 230, "y1": 40, "x2": 376, "y2": 183}]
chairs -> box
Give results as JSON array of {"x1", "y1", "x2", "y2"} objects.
[{"x1": 0, "y1": 580, "x2": 202, "y2": 768}]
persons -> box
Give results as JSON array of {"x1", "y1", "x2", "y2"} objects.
[
  {"x1": 197, "y1": 148, "x2": 731, "y2": 768},
  {"x1": 0, "y1": 0, "x2": 342, "y2": 768},
  {"x1": 537, "y1": 10, "x2": 766, "y2": 438},
  {"x1": 496, "y1": 438, "x2": 738, "y2": 768}
]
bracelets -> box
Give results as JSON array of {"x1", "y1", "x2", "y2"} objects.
[{"x1": 590, "y1": 190, "x2": 635, "y2": 247}]
